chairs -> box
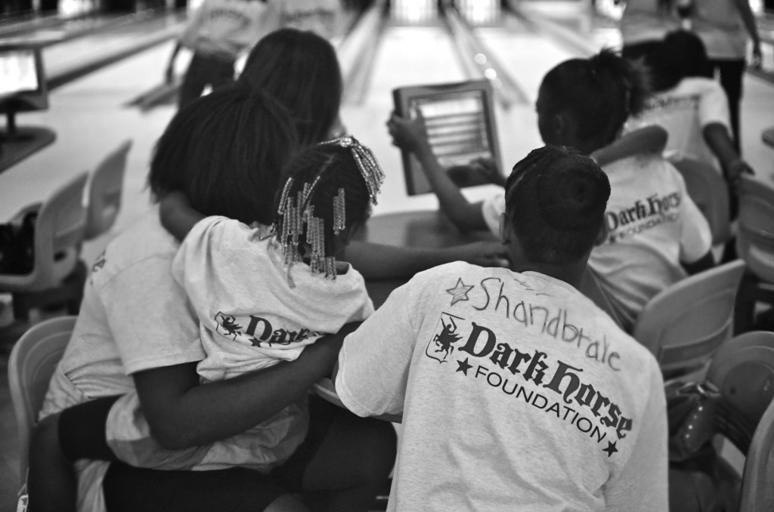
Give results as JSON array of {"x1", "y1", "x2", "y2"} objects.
[
  {"x1": 7, "y1": 316, "x2": 78, "y2": 494},
  {"x1": 0, "y1": 140, "x2": 135, "y2": 294},
  {"x1": 734, "y1": 171, "x2": 774, "y2": 336},
  {"x1": 669, "y1": 153, "x2": 732, "y2": 253},
  {"x1": 632, "y1": 257, "x2": 749, "y2": 397},
  {"x1": 738, "y1": 396, "x2": 774, "y2": 510},
  {"x1": 667, "y1": 329, "x2": 772, "y2": 510}
]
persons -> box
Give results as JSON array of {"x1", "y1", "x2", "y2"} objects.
[
  {"x1": 40, "y1": 27, "x2": 506, "y2": 511},
  {"x1": 384, "y1": 28, "x2": 744, "y2": 332},
  {"x1": 619, "y1": 0, "x2": 764, "y2": 175},
  {"x1": 165, "y1": 1, "x2": 265, "y2": 107},
  {"x1": 329, "y1": 145, "x2": 670, "y2": 512}
]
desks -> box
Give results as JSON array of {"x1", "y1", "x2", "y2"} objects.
[{"x1": 307, "y1": 210, "x2": 504, "y2": 422}]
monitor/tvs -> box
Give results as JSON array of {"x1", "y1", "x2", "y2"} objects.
[{"x1": 1, "y1": 44, "x2": 49, "y2": 115}]
